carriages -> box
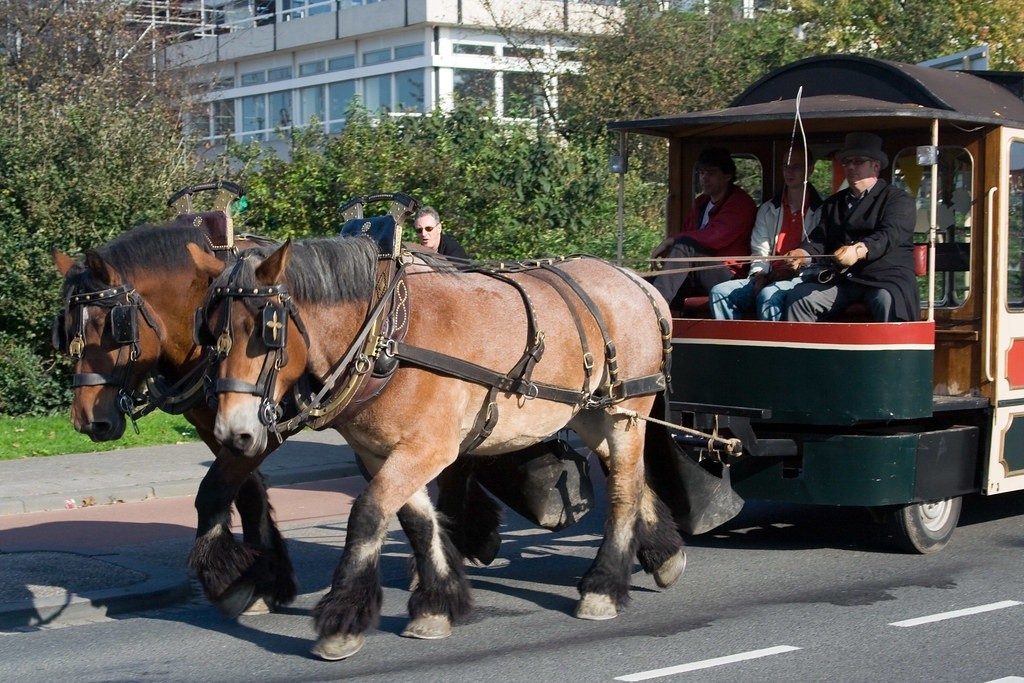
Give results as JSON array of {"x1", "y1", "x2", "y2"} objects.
[{"x1": 52, "y1": 40, "x2": 1024, "y2": 660}]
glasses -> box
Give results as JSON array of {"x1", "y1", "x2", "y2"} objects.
[
  {"x1": 838, "y1": 158, "x2": 873, "y2": 166},
  {"x1": 695, "y1": 169, "x2": 723, "y2": 176},
  {"x1": 415, "y1": 223, "x2": 439, "y2": 233}
]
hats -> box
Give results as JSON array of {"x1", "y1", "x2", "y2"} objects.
[{"x1": 834, "y1": 131, "x2": 889, "y2": 170}]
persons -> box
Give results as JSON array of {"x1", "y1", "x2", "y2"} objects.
[
  {"x1": 411, "y1": 205, "x2": 470, "y2": 268},
  {"x1": 781, "y1": 132, "x2": 922, "y2": 322},
  {"x1": 708, "y1": 144, "x2": 821, "y2": 322},
  {"x1": 641, "y1": 148, "x2": 755, "y2": 312}
]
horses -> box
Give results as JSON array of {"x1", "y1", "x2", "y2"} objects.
[{"x1": 50, "y1": 214, "x2": 687, "y2": 662}]
